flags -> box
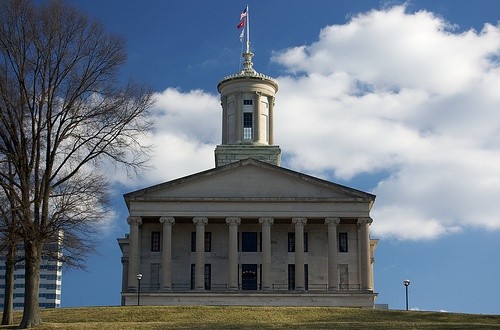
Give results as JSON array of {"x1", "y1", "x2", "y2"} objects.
[
  {"x1": 236, "y1": 16, "x2": 245, "y2": 29},
  {"x1": 239, "y1": 28, "x2": 244, "y2": 43},
  {"x1": 239, "y1": 7, "x2": 247, "y2": 20}
]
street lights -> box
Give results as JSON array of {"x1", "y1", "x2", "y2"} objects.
[
  {"x1": 136, "y1": 274, "x2": 142, "y2": 305},
  {"x1": 404, "y1": 280, "x2": 411, "y2": 310}
]
